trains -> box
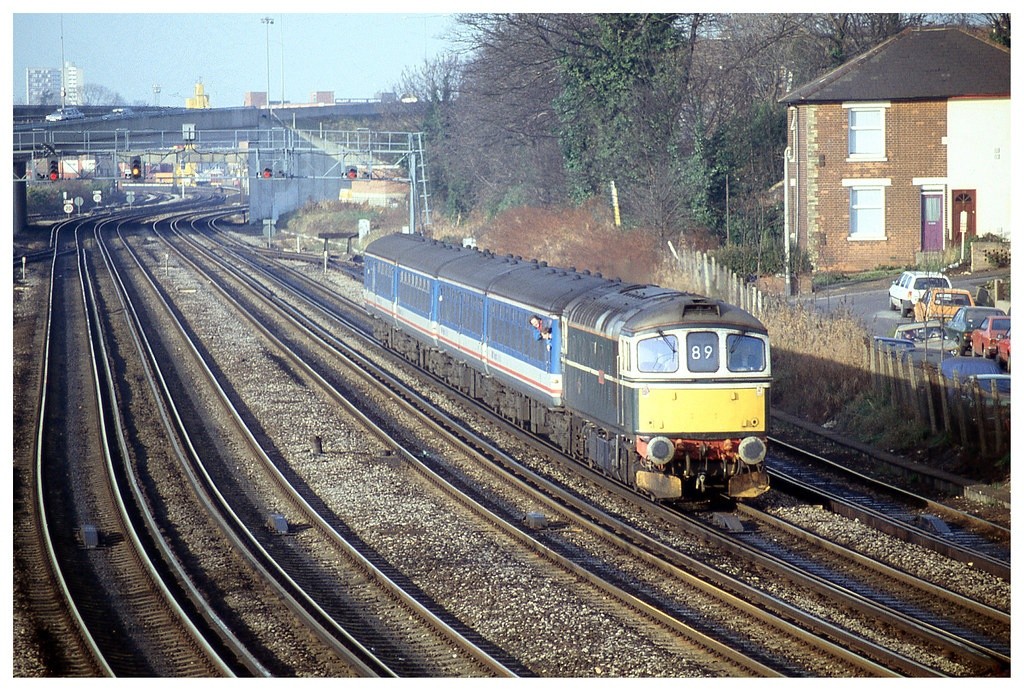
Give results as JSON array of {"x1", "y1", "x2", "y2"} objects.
[{"x1": 363, "y1": 231, "x2": 774, "y2": 506}]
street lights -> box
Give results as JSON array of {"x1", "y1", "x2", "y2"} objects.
[{"x1": 260, "y1": 15, "x2": 275, "y2": 107}]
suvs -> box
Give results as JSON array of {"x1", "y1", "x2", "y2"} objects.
[{"x1": 888, "y1": 271, "x2": 953, "y2": 317}]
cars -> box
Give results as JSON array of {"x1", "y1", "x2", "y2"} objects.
[
  {"x1": 893, "y1": 319, "x2": 962, "y2": 359},
  {"x1": 111, "y1": 108, "x2": 134, "y2": 116},
  {"x1": 961, "y1": 372, "x2": 1011, "y2": 424},
  {"x1": 871, "y1": 335, "x2": 1002, "y2": 385},
  {"x1": 995, "y1": 328, "x2": 1011, "y2": 374},
  {"x1": 45, "y1": 107, "x2": 85, "y2": 122},
  {"x1": 943, "y1": 305, "x2": 1006, "y2": 357},
  {"x1": 910, "y1": 288, "x2": 976, "y2": 325},
  {"x1": 969, "y1": 314, "x2": 1010, "y2": 361}
]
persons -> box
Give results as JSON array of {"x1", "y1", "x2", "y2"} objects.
[{"x1": 529, "y1": 314, "x2": 552, "y2": 351}]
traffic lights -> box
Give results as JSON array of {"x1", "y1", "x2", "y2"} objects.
[
  {"x1": 46, "y1": 156, "x2": 60, "y2": 180},
  {"x1": 345, "y1": 165, "x2": 358, "y2": 178},
  {"x1": 263, "y1": 167, "x2": 271, "y2": 178},
  {"x1": 129, "y1": 156, "x2": 142, "y2": 181}
]
